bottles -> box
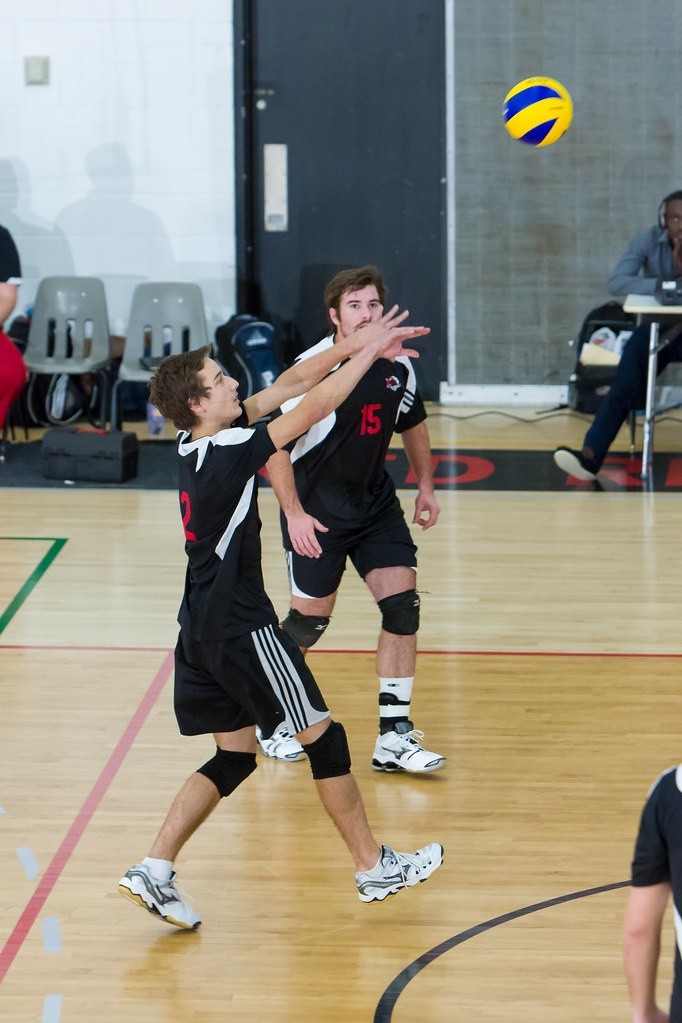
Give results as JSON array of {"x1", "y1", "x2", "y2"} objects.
[{"x1": 147, "y1": 402, "x2": 164, "y2": 435}]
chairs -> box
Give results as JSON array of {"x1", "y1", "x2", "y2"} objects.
[{"x1": 22, "y1": 277, "x2": 212, "y2": 433}]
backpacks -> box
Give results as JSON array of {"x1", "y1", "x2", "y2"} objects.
[
  {"x1": 568, "y1": 301, "x2": 646, "y2": 414},
  {"x1": 215, "y1": 313, "x2": 282, "y2": 425}
]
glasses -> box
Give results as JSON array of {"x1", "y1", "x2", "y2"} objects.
[{"x1": 664, "y1": 214, "x2": 682, "y2": 223}]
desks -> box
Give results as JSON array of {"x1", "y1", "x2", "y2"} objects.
[{"x1": 623, "y1": 294, "x2": 682, "y2": 479}]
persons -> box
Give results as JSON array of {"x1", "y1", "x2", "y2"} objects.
[
  {"x1": 622, "y1": 763, "x2": 682, "y2": 1023},
  {"x1": 255, "y1": 270, "x2": 442, "y2": 773},
  {"x1": 118, "y1": 305, "x2": 444, "y2": 931},
  {"x1": 0, "y1": 225, "x2": 25, "y2": 458},
  {"x1": 552, "y1": 190, "x2": 682, "y2": 481}
]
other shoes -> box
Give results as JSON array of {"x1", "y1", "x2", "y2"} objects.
[{"x1": 553, "y1": 447, "x2": 605, "y2": 480}]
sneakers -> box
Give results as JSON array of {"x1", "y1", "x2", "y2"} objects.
[
  {"x1": 118, "y1": 863, "x2": 202, "y2": 930},
  {"x1": 255, "y1": 721, "x2": 307, "y2": 762},
  {"x1": 371, "y1": 721, "x2": 447, "y2": 773},
  {"x1": 355, "y1": 842, "x2": 444, "y2": 903}
]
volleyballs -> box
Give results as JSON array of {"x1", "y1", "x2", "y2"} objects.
[{"x1": 502, "y1": 77, "x2": 576, "y2": 150}]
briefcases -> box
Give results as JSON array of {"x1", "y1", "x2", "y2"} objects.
[{"x1": 42, "y1": 427, "x2": 139, "y2": 484}]
